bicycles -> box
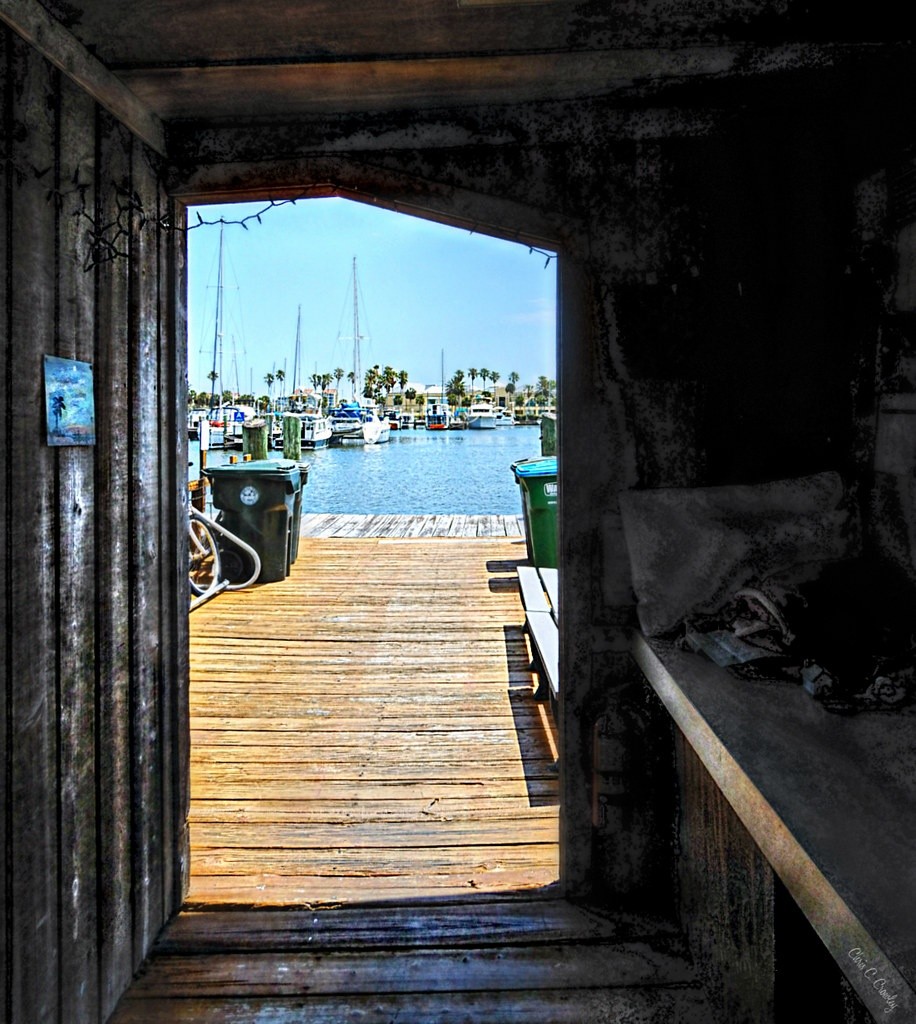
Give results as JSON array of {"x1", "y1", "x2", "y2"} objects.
[{"x1": 187, "y1": 461, "x2": 223, "y2": 597}]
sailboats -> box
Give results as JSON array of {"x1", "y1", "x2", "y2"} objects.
[{"x1": 188, "y1": 213, "x2": 517, "y2": 449}]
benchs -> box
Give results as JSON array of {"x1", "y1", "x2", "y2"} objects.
[{"x1": 506, "y1": 563, "x2": 558, "y2": 772}]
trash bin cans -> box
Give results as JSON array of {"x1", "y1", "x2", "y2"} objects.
[
  {"x1": 507, "y1": 456, "x2": 560, "y2": 568},
  {"x1": 209, "y1": 457, "x2": 309, "y2": 585}
]
